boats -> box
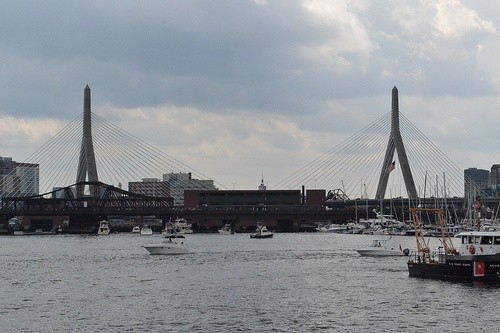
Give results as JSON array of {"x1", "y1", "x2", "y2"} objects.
[
  {"x1": 315, "y1": 222, "x2": 365, "y2": 233},
  {"x1": 407, "y1": 207, "x2": 500, "y2": 285},
  {"x1": 97, "y1": 219, "x2": 111, "y2": 235},
  {"x1": 356, "y1": 237, "x2": 410, "y2": 257},
  {"x1": 161, "y1": 216, "x2": 193, "y2": 238},
  {"x1": 141, "y1": 238, "x2": 195, "y2": 256},
  {"x1": 133, "y1": 224, "x2": 153, "y2": 235},
  {"x1": 217, "y1": 224, "x2": 236, "y2": 235},
  {"x1": 249, "y1": 224, "x2": 274, "y2": 238}
]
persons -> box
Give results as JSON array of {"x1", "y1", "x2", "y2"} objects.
[{"x1": 379, "y1": 242, "x2": 381, "y2": 245}]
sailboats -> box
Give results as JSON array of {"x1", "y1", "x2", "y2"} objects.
[{"x1": 353, "y1": 169, "x2": 500, "y2": 237}]
